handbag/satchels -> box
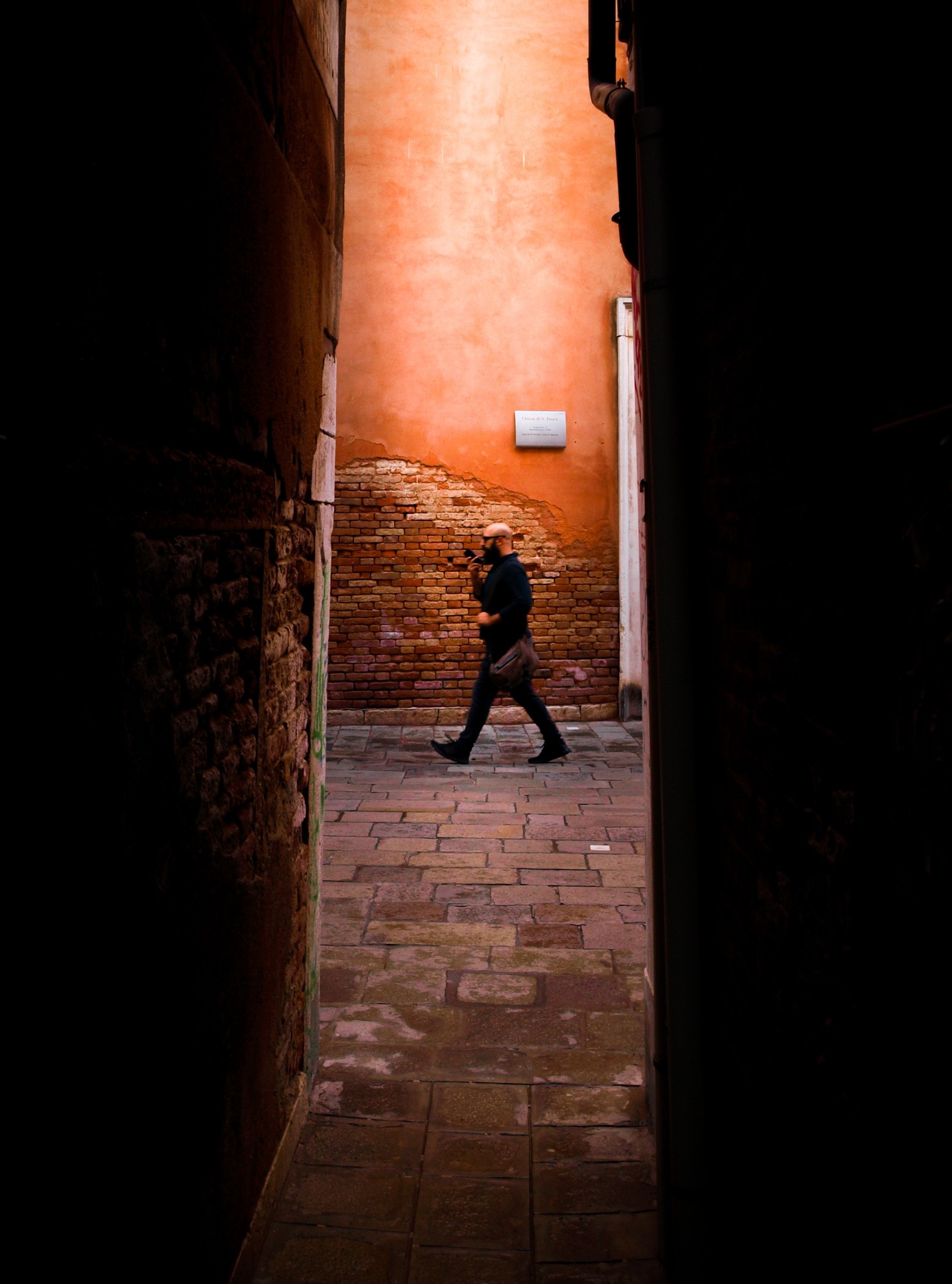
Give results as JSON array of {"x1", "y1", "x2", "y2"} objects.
[{"x1": 489, "y1": 636, "x2": 539, "y2": 693}]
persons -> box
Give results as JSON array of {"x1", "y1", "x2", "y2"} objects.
[{"x1": 430, "y1": 522, "x2": 571, "y2": 765}]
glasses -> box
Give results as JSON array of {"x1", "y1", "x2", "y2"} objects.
[{"x1": 483, "y1": 535, "x2": 505, "y2": 542}]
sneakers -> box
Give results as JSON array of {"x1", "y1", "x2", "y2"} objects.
[
  {"x1": 528, "y1": 738, "x2": 571, "y2": 763},
  {"x1": 431, "y1": 734, "x2": 474, "y2": 764}
]
